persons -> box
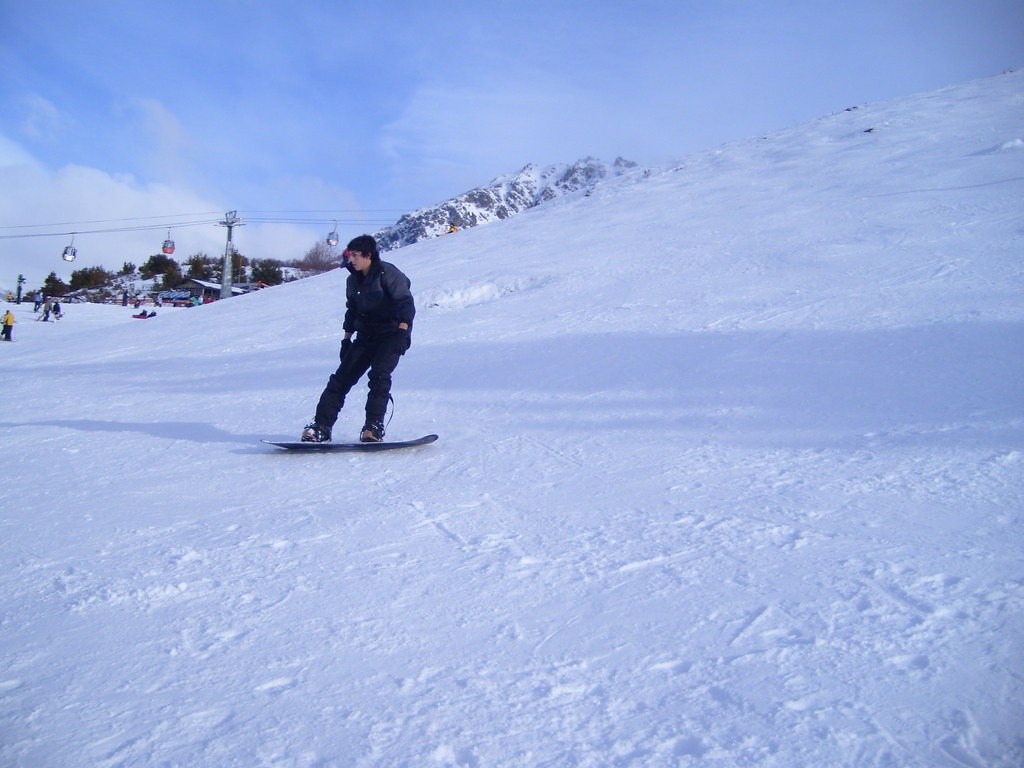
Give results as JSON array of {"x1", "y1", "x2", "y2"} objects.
[
  {"x1": 448, "y1": 222, "x2": 457, "y2": 234},
  {"x1": 301, "y1": 234, "x2": 416, "y2": 442},
  {"x1": 0, "y1": 290, "x2": 215, "y2": 342}
]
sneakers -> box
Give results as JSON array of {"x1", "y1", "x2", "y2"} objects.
[
  {"x1": 301, "y1": 421, "x2": 332, "y2": 443},
  {"x1": 360, "y1": 419, "x2": 385, "y2": 442}
]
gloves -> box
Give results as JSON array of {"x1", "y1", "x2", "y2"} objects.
[
  {"x1": 391, "y1": 328, "x2": 411, "y2": 356},
  {"x1": 340, "y1": 338, "x2": 353, "y2": 362}
]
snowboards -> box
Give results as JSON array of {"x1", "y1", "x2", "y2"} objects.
[
  {"x1": 35, "y1": 319, "x2": 55, "y2": 323},
  {"x1": 133, "y1": 315, "x2": 145, "y2": 318},
  {"x1": 260, "y1": 433, "x2": 439, "y2": 451}
]
